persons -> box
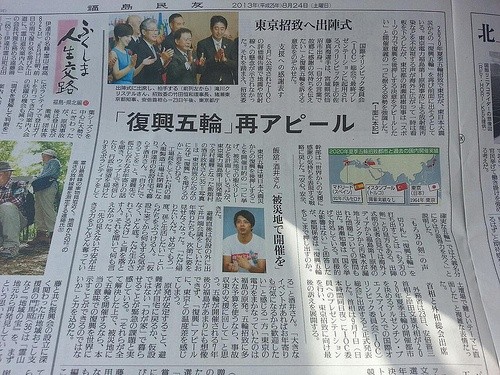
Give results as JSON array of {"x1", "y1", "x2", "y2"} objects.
[
  {"x1": 223, "y1": 208, "x2": 266, "y2": 273},
  {"x1": 108, "y1": 11, "x2": 237, "y2": 85},
  {"x1": 0, "y1": 159, "x2": 34, "y2": 262},
  {"x1": 24, "y1": 147, "x2": 62, "y2": 246}
]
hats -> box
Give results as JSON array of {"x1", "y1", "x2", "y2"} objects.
[
  {"x1": 41, "y1": 149, "x2": 58, "y2": 158},
  {"x1": 0, "y1": 161, "x2": 14, "y2": 173}
]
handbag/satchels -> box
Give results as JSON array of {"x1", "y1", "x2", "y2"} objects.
[{"x1": 28, "y1": 174, "x2": 51, "y2": 191}]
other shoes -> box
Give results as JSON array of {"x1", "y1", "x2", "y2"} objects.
[
  {"x1": 1, "y1": 247, "x2": 20, "y2": 258},
  {"x1": 26, "y1": 231, "x2": 45, "y2": 245}
]
